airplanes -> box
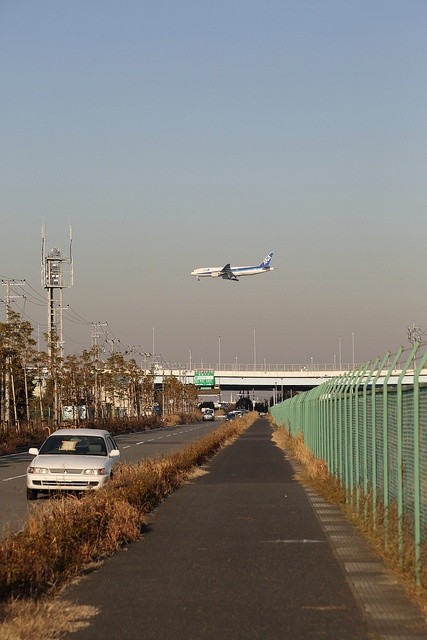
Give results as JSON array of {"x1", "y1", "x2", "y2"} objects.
[{"x1": 190, "y1": 252, "x2": 274, "y2": 281}]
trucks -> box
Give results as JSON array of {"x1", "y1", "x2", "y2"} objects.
[{"x1": 201, "y1": 407, "x2": 215, "y2": 422}]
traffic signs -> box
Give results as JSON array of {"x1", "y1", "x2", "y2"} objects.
[{"x1": 194, "y1": 370, "x2": 215, "y2": 386}]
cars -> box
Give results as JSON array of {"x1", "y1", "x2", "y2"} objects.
[
  {"x1": 26, "y1": 429, "x2": 121, "y2": 500},
  {"x1": 224, "y1": 411, "x2": 242, "y2": 421}
]
